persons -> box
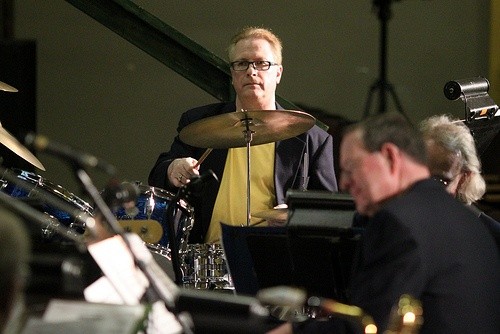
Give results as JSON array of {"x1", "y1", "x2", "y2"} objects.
[
  {"x1": 418, "y1": 113, "x2": 500, "y2": 247},
  {"x1": 148, "y1": 29, "x2": 338, "y2": 255},
  {"x1": 267, "y1": 115, "x2": 500, "y2": 334}
]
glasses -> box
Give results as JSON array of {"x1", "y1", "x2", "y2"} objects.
[
  {"x1": 229, "y1": 60, "x2": 278, "y2": 71},
  {"x1": 432, "y1": 173, "x2": 462, "y2": 186}
]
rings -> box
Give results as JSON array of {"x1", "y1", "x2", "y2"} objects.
[{"x1": 177, "y1": 174, "x2": 182, "y2": 182}]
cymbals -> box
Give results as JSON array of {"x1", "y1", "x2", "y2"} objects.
[
  {"x1": 0, "y1": 121, "x2": 45, "y2": 171},
  {"x1": 0, "y1": 80, "x2": 19, "y2": 92},
  {"x1": 179, "y1": 109, "x2": 316, "y2": 149}
]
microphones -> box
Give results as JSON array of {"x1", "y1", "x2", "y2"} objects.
[{"x1": 25, "y1": 133, "x2": 116, "y2": 175}]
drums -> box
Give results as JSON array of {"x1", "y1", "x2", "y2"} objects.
[
  {"x1": 0, "y1": 168, "x2": 95, "y2": 255},
  {"x1": 179, "y1": 242, "x2": 236, "y2": 291},
  {"x1": 106, "y1": 181, "x2": 194, "y2": 286}
]
